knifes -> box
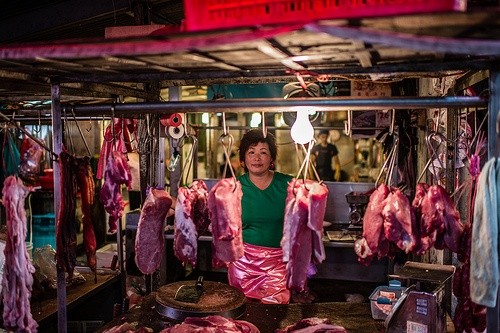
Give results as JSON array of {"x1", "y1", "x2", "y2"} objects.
[{"x1": 175, "y1": 275, "x2": 204, "y2": 303}]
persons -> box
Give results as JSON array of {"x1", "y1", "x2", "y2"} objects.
[
  {"x1": 229, "y1": 129, "x2": 292, "y2": 305},
  {"x1": 311, "y1": 129, "x2": 342, "y2": 181}
]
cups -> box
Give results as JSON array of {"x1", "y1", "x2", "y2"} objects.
[{"x1": 26, "y1": 241, "x2": 33, "y2": 261}]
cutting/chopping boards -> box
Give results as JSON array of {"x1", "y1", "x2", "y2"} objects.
[{"x1": 154, "y1": 280, "x2": 247, "y2": 323}]
